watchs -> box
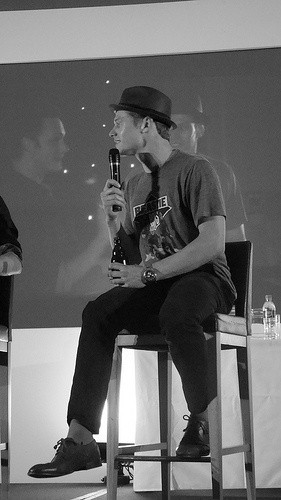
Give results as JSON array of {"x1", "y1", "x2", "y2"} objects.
[{"x1": 143, "y1": 262, "x2": 158, "y2": 285}]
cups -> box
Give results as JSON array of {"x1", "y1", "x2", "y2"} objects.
[
  {"x1": 251, "y1": 309, "x2": 263, "y2": 337},
  {"x1": 277, "y1": 314, "x2": 281, "y2": 337}
]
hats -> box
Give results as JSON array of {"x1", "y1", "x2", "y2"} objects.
[
  {"x1": 110, "y1": 86, "x2": 178, "y2": 130},
  {"x1": 170, "y1": 92, "x2": 215, "y2": 125}
]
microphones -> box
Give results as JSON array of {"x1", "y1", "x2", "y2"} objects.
[{"x1": 109, "y1": 148, "x2": 123, "y2": 212}]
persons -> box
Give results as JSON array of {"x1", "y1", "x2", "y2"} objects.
[
  {"x1": 2, "y1": 93, "x2": 258, "y2": 326},
  {"x1": 1, "y1": 197, "x2": 24, "y2": 277},
  {"x1": 29, "y1": 85, "x2": 235, "y2": 478}
]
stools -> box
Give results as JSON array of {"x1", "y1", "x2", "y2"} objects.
[{"x1": 106, "y1": 241, "x2": 256, "y2": 500}]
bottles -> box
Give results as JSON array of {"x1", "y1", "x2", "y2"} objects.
[
  {"x1": 263, "y1": 295, "x2": 277, "y2": 339},
  {"x1": 111, "y1": 237, "x2": 126, "y2": 279}
]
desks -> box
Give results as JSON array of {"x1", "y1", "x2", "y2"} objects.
[{"x1": 133, "y1": 339, "x2": 281, "y2": 500}]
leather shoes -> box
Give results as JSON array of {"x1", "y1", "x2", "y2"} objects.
[
  {"x1": 176, "y1": 414, "x2": 210, "y2": 457},
  {"x1": 28, "y1": 438, "x2": 103, "y2": 478}
]
